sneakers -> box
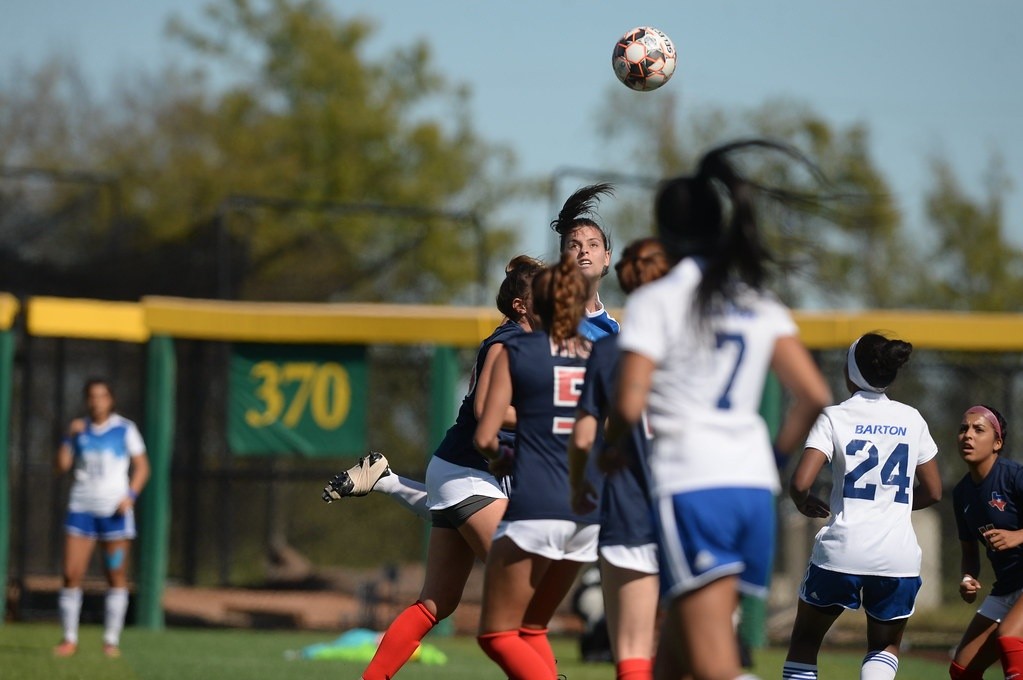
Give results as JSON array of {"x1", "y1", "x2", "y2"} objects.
[{"x1": 321, "y1": 451, "x2": 390, "y2": 504}]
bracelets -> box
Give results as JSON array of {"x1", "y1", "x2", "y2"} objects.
[
  {"x1": 62, "y1": 436, "x2": 73, "y2": 444},
  {"x1": 128, "y1": 490, "x2": 137, "y2": 501},
  {"x1": 963, "y1": 577, "x2": 972, "y2": 582}
]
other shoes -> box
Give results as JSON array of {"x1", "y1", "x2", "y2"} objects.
[
  {"x1": 102, "y1": 644, "x2": 121, "y2": 657},
  {"x1": 52, "y1": 639, "x2": 77, "y2": 656}
]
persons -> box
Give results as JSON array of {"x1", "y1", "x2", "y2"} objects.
[
  {"x1": 321, "y1": 137, "x2": 832, "y2": 680},
  {"x1": 949, "y1": 404, "x2": 1023, "y2": 680},
  {"x1": 782, "y1": 333, "x2": 942, "y2": 680},
  {"x1": 51, "y1": 379, "x2": 152, "y2": 657}
]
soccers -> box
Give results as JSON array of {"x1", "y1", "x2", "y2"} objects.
[{"x1": 611, "y1": 26, "x2": 677, "y2": 92}]
